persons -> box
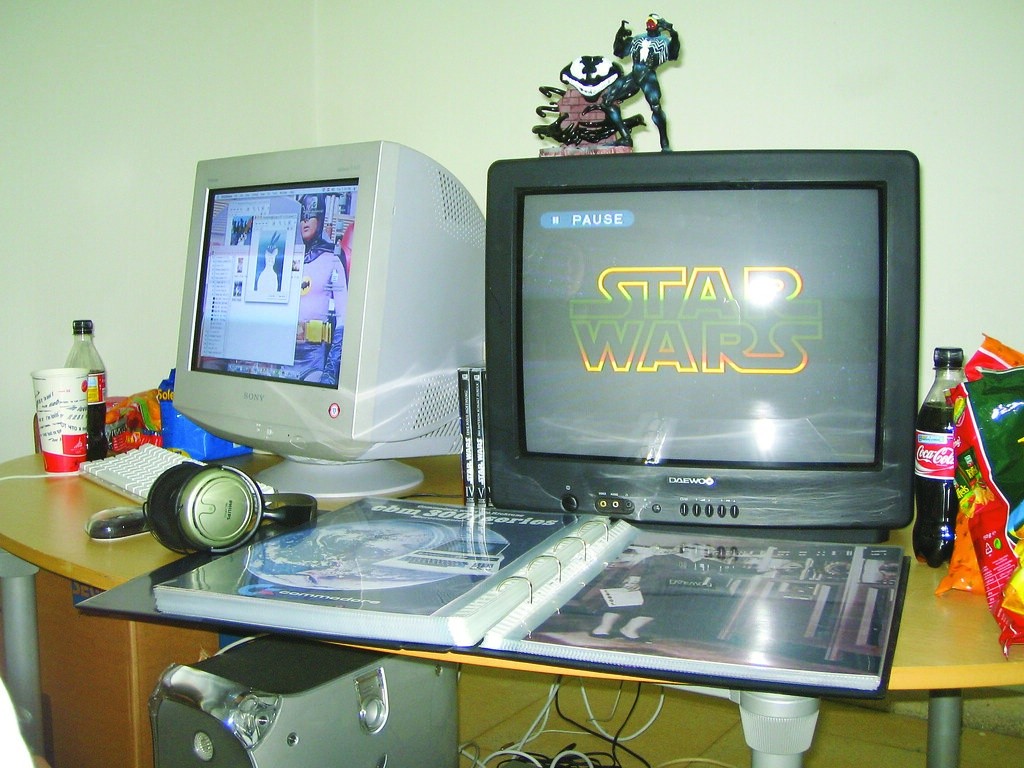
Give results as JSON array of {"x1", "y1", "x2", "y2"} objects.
[
  {"x1": 282, "y1": 193, "x2": 347, "y2": 386},
  {"x1": 591, "y1": 543, "x2": 790, "y2": 640}
]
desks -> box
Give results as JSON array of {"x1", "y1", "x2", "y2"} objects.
[{"x1": 0, "y1": 450, "x2": 1023, "y2": 768}]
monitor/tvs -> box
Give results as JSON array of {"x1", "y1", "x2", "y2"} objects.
[
  {"x1": 485, "y1": 148, "x2": 920, "y2": 546},
  {"x1": 171, "y1": 139, "x2": 488, "y2": 503}
]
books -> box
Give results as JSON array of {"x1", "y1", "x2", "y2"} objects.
[{"x1": 153, "y1": 495, "x2": 911, "y2": 699}]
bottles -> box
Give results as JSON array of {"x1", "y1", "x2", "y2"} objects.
[
  {"x1": 63, "y1": 319, "x2": 109, "y2": 462},
  {"x1": 913, "y1": 347, "x2": 965, "y2": 568}
]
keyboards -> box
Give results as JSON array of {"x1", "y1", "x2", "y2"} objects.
[{"x1": 78, "y1": 442, "x2": 275, "y2": 504}]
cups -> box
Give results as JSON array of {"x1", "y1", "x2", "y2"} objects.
[{"x1": 30, "y1": 367, "x2": 90, "y2": 475}]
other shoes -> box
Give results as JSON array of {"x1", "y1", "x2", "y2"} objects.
[
  {"x1": 616, "y1": 629, "x2": 649, "y2": 643},
  {"x1": 590, "y1": 630, "x2": 612, "y2": 639}
]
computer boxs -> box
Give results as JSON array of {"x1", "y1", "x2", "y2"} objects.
[{"x1": 147, "y1": 633, "x2": 460, "y2": 768}]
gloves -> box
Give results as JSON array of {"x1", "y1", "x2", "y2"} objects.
[{"x1": 320, "y1": 327, "x2": 344, "y2": 384}]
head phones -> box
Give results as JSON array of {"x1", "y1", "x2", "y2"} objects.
[{"x1": 146, "y1": 460, "x2": 317, "y2": 558}]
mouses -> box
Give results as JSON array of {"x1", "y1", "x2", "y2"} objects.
[{"x1": 85, "y1": 506, "x2": 150, "y2": 541}]
